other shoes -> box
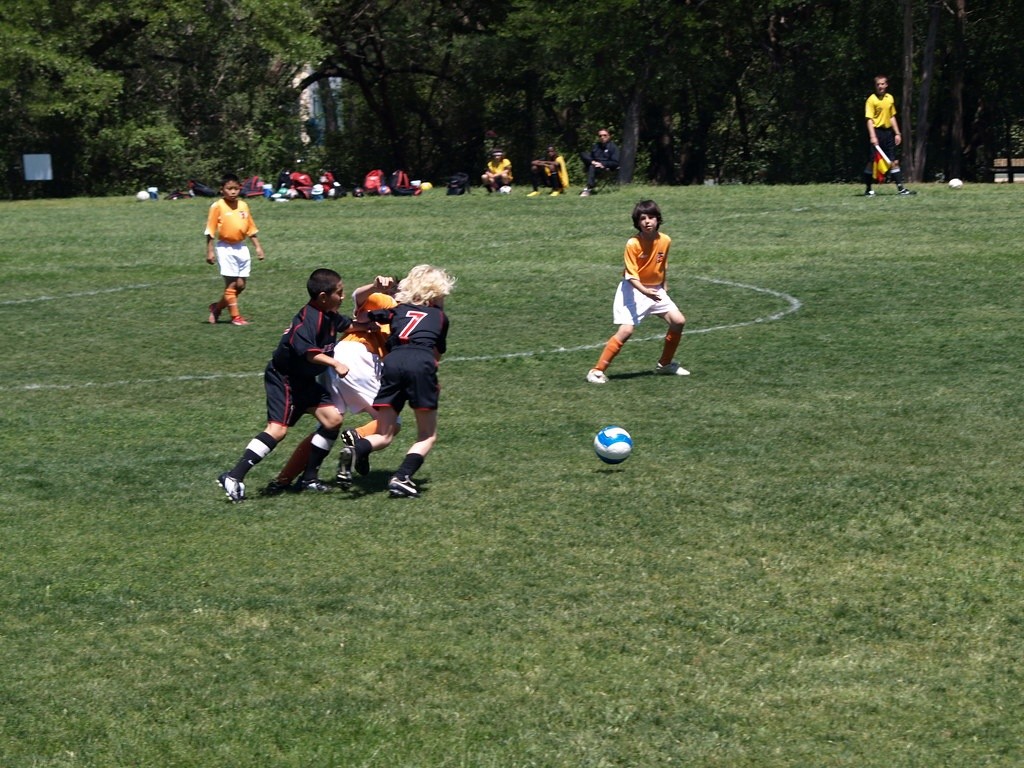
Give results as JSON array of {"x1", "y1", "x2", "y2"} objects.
[
  {"x1": 527, "y1": 191, "x2": 540, "y2": 197},
  {"x1": 550, "y1": 188, "x2": 562, "y2": 196},
  {"x1": 580, "y1": 189, "x2": 591, "y2": 196}
]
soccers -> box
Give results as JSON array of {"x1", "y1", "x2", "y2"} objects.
[
  {"x1": 378, "y1": 185, "x2": 391, "y2": 195},
  {"x1": 949, "y1": 178, "x2": 963, "y2": 190},
  {"x1": 500, "y1": 186, "x2": 512, "y2": 194},
  {"x1": 136, "y1": 191, "x2": 150, "y2": 200},
  {"x1": 594, "y1": 425, "x2": 634, "y2": 464}
]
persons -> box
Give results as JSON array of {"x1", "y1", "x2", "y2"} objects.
[
  {"x1": 586, "y1": 201, "x2": 690, "y2": 384},
  {"x1": 216, "y1": 264, "x2": 458, "y2": 505},
  {"x1": 481, "y1": 148, "x2": 514, "y2": 195},
  {"x1": 579, "y1": 128, "x2": 620, "y2": 197},
  {"x1": 204, "y1": 174, "x2": 264, "y2": 325},
  {"x1": 864, "y1": 75, "x2": 916, "y2": 195},
  {"x1": 527, "y1": 147, "x2": 569, "y2": 197}
]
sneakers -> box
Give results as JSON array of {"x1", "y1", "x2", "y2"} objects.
[
  {"x1": 231, "y1": 316, "x2": 248, "y2": 325},
  {"x1": 209, "y1": 302, "x2": 219, "y2": 324},
  {"x1": 259, "y1": 480, "x2": 285, "y2": 495},
  {"x1": 655, "y1": 362, "x2": 690, "y2": 375},
  {"x1": 389, "y1": 475, "x2": 420, "y2": 499},
  {"x1": 336, "y1": 446, "x2": 355, "y2": 492},
  {"x1": 341, "y1": 428, "x2": 361, "y2": 446},
  {"x1": 216, "y1": 472, "x2": 245, "y2": 504},
  {"x1": 293, "y1": 477, "x2": 336, "y2": 493},
  {"x1": 586, "y1": 369, "x2": 606, "y2": 383},
  {"x1": 865, "y1": 191, "x2": 876, "y2": 196},
  {"x1": 898, "y1": 189, "x2": 916, "y2": 194}
]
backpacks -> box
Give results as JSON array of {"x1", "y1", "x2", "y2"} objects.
[
  {"x1": 164, "y1": 169, "x2": 421, "y2": 200},
  {"x1": 446, "y1": 172, "x2": 468, "y2": 195}
]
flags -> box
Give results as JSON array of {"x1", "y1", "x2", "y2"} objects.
[{"x1": 872, "y1": 153, "x2": 889, "y2": 182}]
